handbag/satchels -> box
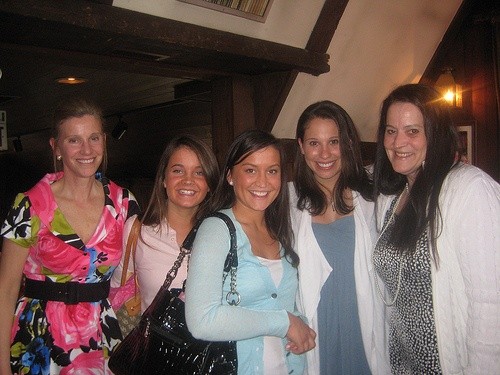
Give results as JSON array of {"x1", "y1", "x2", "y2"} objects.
[
  {"x1": 108, "y1": 211, "x2": 240, "y2": 375},
  {"x1": 108, "y1": 216, "x2": 141, "y2": 342}
]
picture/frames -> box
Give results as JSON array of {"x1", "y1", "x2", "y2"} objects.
[
  {"x1": 456, "y1": 120, "x2": 478, "y2": 168},
  {"x1": 182, "y1": 1, "x2": 274, "y2": 23}
]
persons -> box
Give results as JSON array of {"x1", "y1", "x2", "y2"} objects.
[
  {"x1": 373, "y1": 84, "x2": 500, "y2": 375},
  {"x1": 186, "y1": 131, "x2": 316, "y2": 375},
  {"x1": 0, "y1": 92, "x2": 141, "y2": 375},
  {"x1": 287, "y1": 101, "x2": 396, "y2": 375},
  {"x1": 108, "y1": 134, "x2": 220, "y2": 316}
]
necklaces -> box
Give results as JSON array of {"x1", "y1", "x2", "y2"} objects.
[{"x1": 371, "y1": 182, "x2": 410, "y2": 307}]
